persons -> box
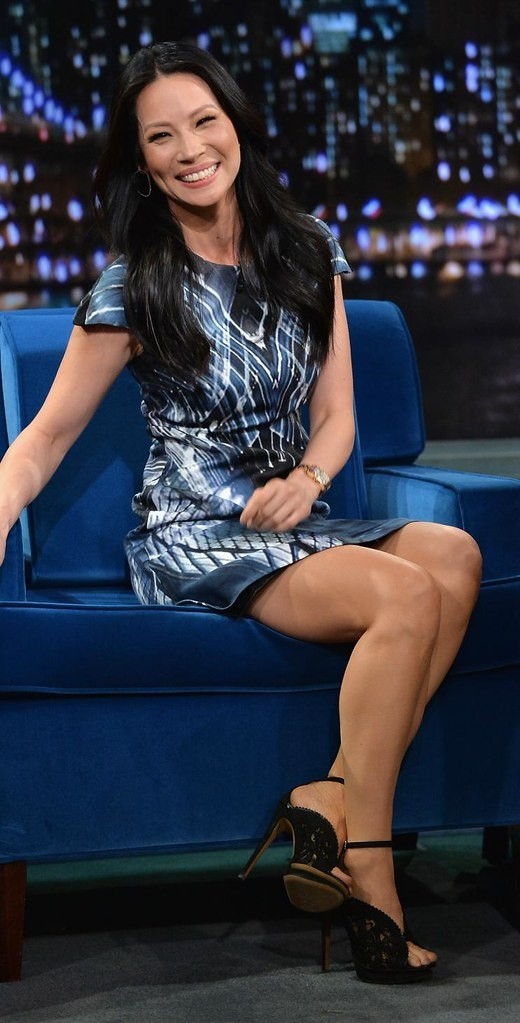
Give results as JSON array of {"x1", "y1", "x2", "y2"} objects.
[{"x1": 0, "y1": 42, "x2": 483, "y2": 986}]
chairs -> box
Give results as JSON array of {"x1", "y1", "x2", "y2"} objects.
[{"x1": 0, "y1": 299, "x2": 520, "y2": 980}]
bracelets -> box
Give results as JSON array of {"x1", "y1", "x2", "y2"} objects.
[{"x1": 290, "y1": 461, "x2": 332, "y2": 500}]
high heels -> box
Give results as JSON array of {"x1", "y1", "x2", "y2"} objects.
[
  {"x1": 238, "y1": 776, "x2": 350, "y2": 913},
  {"x1": 321, "y1": 896, "x2": 435, "y2": 984}
]
